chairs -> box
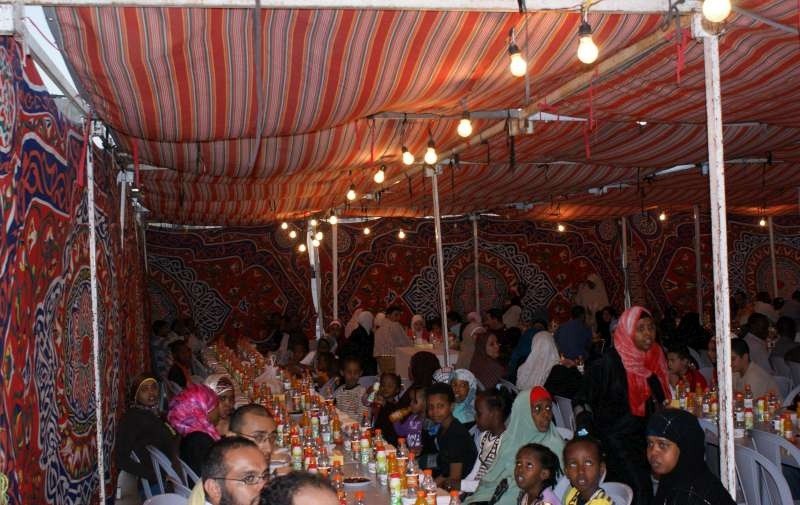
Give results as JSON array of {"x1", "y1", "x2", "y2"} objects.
[
  {"x1": 127, "y1": 377, "x2": 201, "y2": 505},
  {"x1": 551, "y1": 369, "x2": 800, "y2": 505}
]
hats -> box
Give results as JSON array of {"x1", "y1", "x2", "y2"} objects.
[
  {"x1": 530, "y1": 386, "x2": 552, "y2": 402},
  {"x1": 169, "y1": 340, "x2": 193, "y2": 361}
]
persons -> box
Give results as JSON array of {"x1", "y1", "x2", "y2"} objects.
[
  {"x1": 256, "y1": 314, "x2": 341, "y2": 405},
  {"x1": 589, "y1": 306, "x2": 672, "y2": 505},
  {"x1": 344, "y1": 274, "x2": 618, "y2": 505},
  {"x1": 633, "y1": 408, "x2": 739, "y2": 505},
  {"x1": 226, "y1": 404, "x2": 279, "y2": 478},
  {"x1": 259, "y1": 471, "x2": 339, "y2": 505},
  {"x1": 189, "y1": 436, "x2": 269, "y2": 505},
  {"x1": 335, "y1": 363, "x2": 368, "y2": 422},
  {"x1": 117, "y1": 319, "x2": 235, "y2": 505},
  {"x1": 655, "y1": 291, "x2": 800, "y2": 412}
]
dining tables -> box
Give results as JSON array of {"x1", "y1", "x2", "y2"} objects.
[{"x1": 198, "y1": 345, "x2": 449, "y2": 505}]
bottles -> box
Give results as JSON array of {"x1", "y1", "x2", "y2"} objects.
[
  {"x1": 731, "y1": 319, "x2": 742, "y2": 337},
  {"x1": 767, "y1": 327, "x2": 776, "y2": 352},
  {"x1": 216, "y1": 335, "x2": 463, "y2": 505},
  {"x1": 576, "y1": 356, "x2": 584, "y2": 375},
  {"x1": 674, "y1": 375, "x2": 800, "y2": 444}
]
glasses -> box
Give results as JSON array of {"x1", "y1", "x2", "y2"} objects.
[
  {"x1": 235, "y1": 432, "x2": 279, "y2": 445},
  {"x1": 217, "y1": 474, "x2": 271, "y2": 485}
]
